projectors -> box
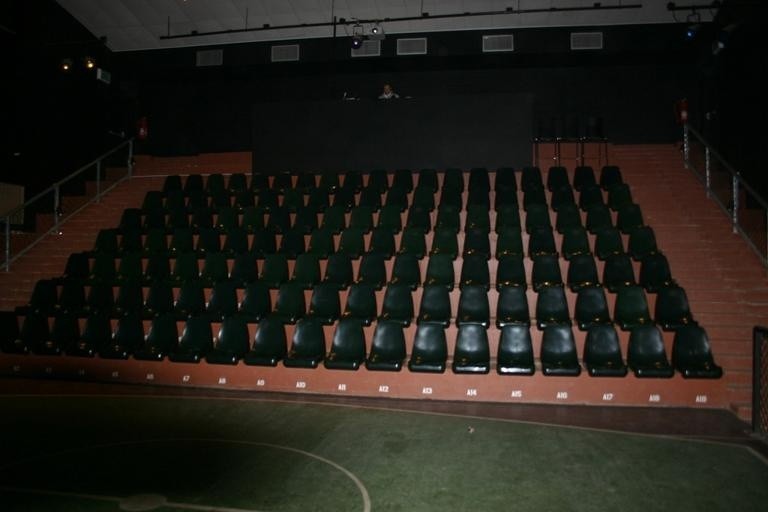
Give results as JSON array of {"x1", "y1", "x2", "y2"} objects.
[{"x1": 367, "y1": 26, "x2": 386, "y2": 38}]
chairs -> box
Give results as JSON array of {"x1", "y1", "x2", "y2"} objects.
[
  {"x1": 95, "y1": 228, "x2": 116, "y2": 254},
  {"x1": 275, "y1": 280, "x2": 305, "y2": 323},
  {"x1": 54, "y1": 253, "x2": 89, "y2": 283},
  {"x1": 358, "y1": 188, "x2": 381, "y2": 213},
  {"x1": 258, "y1": 188, "x2": 279, "y2": 214},
  {"x1": 385, "y1": 187, "x2": 408, "y2": 213},
  {"x1": 90, "y1": 251, "x2": 115, "y2": 283},
  {"x1": 429, "y1": 225, "x2": 459, "y2": 260},
  {"x1": 309, "y1": 280, "x2": 340, "y2": 325},
  {"x1": 442, "y1": 169, "x2": 464, "y2": 194},
  {"x1": 458, "y1": 253, "x2": 490, "y2": 290},
  {"x1": 405, "y1": 205, "x2": 431, "y2": 234},
  {"x1": 168, "y1": 315, "x2": 212, "y2": 363},
  {"x1": 171, "y1": 248, "x2": 199, "y2": 286},
  {"x1": 94, "y1": 313, "x2": 143, "y2": 359},
  {"x1": 282, "y1": 187, "x2": 304, "y2": 213},
  {"x1": 495, "y1": 253, "x2": 528, "y2": 293},
  {"x1": 497, "y1": 325, "x2": 536, "y2": 376},
  {"x1": 583, "y1": 325, "x2": 628, "y2": 377},
  {"x1": 464, "y1": 203, "x2": 491, "y2": 234},
  {"x1": 308, "y1": 228, "x2": 335, "y2": 259},
  {"x1": 595, "y1": 225, "x2": 626, "y2": 261},
  {"x1": 378, "y1": 204, "x2": 402, "y2": 235},
  {"x1": 389, "y1": 251, "x2": 421, "y2": 290},
  {"x1": 320, "y1": 173, "x2": 340, "y2": 194},
  {"x1": 528, "y1": 225, "x2": 559, "y2": 261},
  {"x1": 116, "y1": 250, "x2": 146, "y2": 285},
  {"x1": 608, "y1": 182, "x2": 632, "y2": 211},
  {"x1": 525, "y1": 204, "x2": 551, "y2": 234},
  {"x1": 536, "y1": 286, "x2": 572, "y2": 330},
  {"x1": 333, "y1": 186, "x2": 354, "y2": 213},
  {"x1": 547, "y1": 166, "x2": 569, "y2": 191},
  {"x1": 260, "y1": 254, "x2": 289, "y2": 289},
  {"x1": 574, "y1": 285, "x2": 612, "y2": 331},
  {"x1": 192, "y1": 208, "x2": 214, "y2": 233},
  {"x1": 226, "y1": 172, "x2": 246, "y2": 195},
  {"x1": 616, "y1": 203, "x2": 643, "y2": 235},
  {"x1": 377, "y1": 280, "x2": 413, "y2": 328},
  {"x1": 524, "y1": 183, "x2": 547, "y2": 211},
  {"x1": 434, "y1": 205, "x2": 460, "y2": 232},
  {"x1": 602, "y1": 254, "x2": 636, "y2": 293},
  {"x1": 350, "y1": 205, "x2": 373, "y2": 234},
  {"x1": 417, "y1": 169, "x2": 438, "y2": 194},
  {"x1": 455, "y1": 281, "x2": 491, "y2": 329},
  {"x1": 205, "y1": 173, "x2": 223, "y2": 195},
  {"x1": 627, "y1": 325, "x2": 674, "y2": 378},
  {"x1": 366, "y1": 321, "x2": 406, "y2": 372},
  {"x1": 323, "y1": 252, "x2": 354, "y2": 290},
  {"x1": 164, "y1": 194, "x2": 185, "y2": 212},
  {"x1": 321, "y1": 205, "x2": 345, "y2": 234},
  {"x1": 233, "y1": 189, "x2": 254, "y2": 213},
  {"x1": 495, "y1": 286, "x2": 531, "y2": 329},
  {"x1": 392, "y1": 171, "x2": 413, "y2": 194},
  {"x1": 369, "y1": 224, "x2": 394, "y2": 260},
  {"x1": 324, "y1": 317, "x2": 367, "y2": 370},
  {"x1": 86, "y1": 284, "x2": 114, "y2": 317},
  {"x1": 23, "y1": 310, "x2": 51, "y2": 354},
  {"x1": 337, "y1": 228, "x2": 365, "y2": 260},
  {"x1": 241, "y1": 206, "x2": 264, "y2": 234},
  {"x1": 231, "y1": 251, "x2": 258, "y2": 288},
  {"x1": 82, "y1": 314, "x2": 112, "y2": 356},
  {"x1": 540, "y1": 322, "x2": 581, "y2": 377},
  {"x1": 216, "y1": 206, "x2": 239, "y2": 233},
  {"x1": 0, "y1": 311, "x2": 22, "y2": 351},
  {"x1": 397, "y1": 225, "x2": 427, "y2": 260},
  {"x1": 639, "y1": 254, "x2": 674, "y2": 293},
  {"x1": 462, "y1": 224, "x2": 491, "y2": 260},
  {"x1": 521, "y1": 166, "x2": 545, "y2": 191},
  {"x1": 344, "y1": 172, "x2": 364, "y2": 195},
  {"x1": 144, "y1": 284, "x2": 174, "y2": 319},
  {"x1": 293, "y1": 206, "x2": 318, "y2": 234},
  {"x1": 600, "y1": 166, "x2": 622, "y2": 192},
  {"x1": 240, "y1": 279, "x2": 271, "y2": 322},
  {"x1": 495, "y1": 203, "x2": 521, "y2": 233},
  {"x1": 169, "y1": 226, "x2": 195, "y2": 256},
  {"x1": 579, "y1": 183, "x2": 604, "y2": 211},
  {"x1": 283, "y1": 316, "x2": 325, "y2": 369},
  {"x1": 292, "y1": 252, "x2": 320, "y2": 289},
  {"x1": 205, "y1": 311, "x2": 250, "y2": 366},
  {"x1": 466, "y1": 185, "x2": 490, "y2": 211},
  {"x1": 585, "y1": 203, "x2": 612, "y2": 235},
  {"x1": 144, "y1": 209, "x2": 165, "y2": 235},
  {"x1": 493, "y1": 168, "x2": 517, "y2": 192},
  {"x1": 211, "y1": 192, "x2": 230, "y2": 212},
  {"x1": 250, "y1": 226, "x2": 277, "y2": 259},
  {"x1": 467, "y1": 167, "x2": 490, "y2": 191},
  {"x1": 113, "y1": 280, "x2": 142, "y2": 316},
  {"x1": 657, "y1": 285, "x2": 699, "y2": 331},
  {"x1": 531, "y1": 255, "x2": 565, "y2": 293},
  {"x1": 174, "y1": 283, "x2": 206, "y2": 319},
  {"x1": 367, "y1": 172, "x2": 388, "y2": 194},
  {"x1": 494, "y1": 188, "x2": 519, "y2": 212},
  {"x1": 615, "y1": 286, "x2": 655, "y2": 330},
  {"x1": 55, "y1": 284, "x2": 85, "y2": 316},
  {"x1": 266, "y1": 207, "x2": 290, "y2": 234},
  {"x1": 296, "y1": 173, "x2": 317, "y2": 195},
  {"x1": 556, "y1": 203, "x2": 582, "y2": 234},
  {"x1": 115, "y1": 208, "x2": 140, "y2": 234},
  {"x1": 551, "y1": 185, "x2": 575, "y2": 211},
  {"x1": 561, "y1": 225, "x2": 592, "y2": 260},
  {"x1": 145, "y1": 229, "x2": 165, "y2": 256},
  {"x1": 407, "y1": 322, "x2": 447, "y2": 373},
  {"x1": 628, "y1": 224, "x2": 660, "y2": 261},
  {"x1": 184, "y1": 174, "x2": 203, "y2": 196},
  {"x1": 278, "y1": 225, "x2": 305, "y2": 258},
  {"x1": 14, "y1": 279, "x2": 57, "y2": 317},
  {"x1": 452, "y1": 325, "x2": 490, "y2": 374},
  {"x1": 416, "y1": 282, "x2": 451, "y2": 328},
  {"x1": 423, "y1": 253, "x2": 454, "y2": 291},
  {"x1": 495, "y1": 226, "x2": 524, "y2": 260},
  {"x1": 357, "y1": 250, "x2": 386, "y2": 290},
  {"x1": 201, "y1": 249, "x2": 228, "y2": 288},
  {"x1": 188, "y1": 194, "x2": 206, "y2": 212},
  {"x1": 412, "y1": 187, "x2": 435, "y2": 212},
  {"x1": 344, "y1": 281, "x2": 378, "y2": 327},
  {"x1": 272, "y1": 172, "x2": 292, "y2": 196},
  {"x1": 118, "y1": 230, "x2": 144, "y2": 257},
  {"x1": 567, "y1": 254, "x2": 600, "y2": 293},
  {"x1": 162, "y1": 174, "x2": 181, "y2": 196},
  {"x1": 144, "y1": 248, "x2": 170, "y2": 285},
  {"x1": 574, "y1": 165, "x2": 595, "y2": 192},
  {"x1": 166, "y1": 207, "x2": 188, "y2": 234},
  {"x1": 142, "y1": 190, "x2": 163, "y2": 210},
  {"x1": 250, "y1": 172, "x2": 269, "y2": 195},
  {"x1": 51, "y1": 314, "x2": 78, "y2": 352},
  {"x1": 243, "y1": 312, "x2": 287, "y2": 367},
  {"x1": 223, "y1": 227, "x2": 248, "y2": 257},
  {"x1": 438, "y1": 187, "x2": 462, "y2": 211},
  {"x1": 206, "y1": 279, "x2": 237, "y2": 320},
  {"x1": 671, "y1": 324, "x2": 723, "y2": 379},
  {"x1": 307, "y1": 187, "x2": 330, "y2": 213},
  {"x1": 133, "y1": 311, "x2": 178, "y2": 361}
]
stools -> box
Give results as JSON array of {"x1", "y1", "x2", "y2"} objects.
[
  {"x1": 581, "y1": 136, "x2": 608, "y2": 168},
  {"x1": 556, "y1": 137, "x2": 580, "y2": 168},
  {"x1": 197, "y1": 225, "x2": 221, "y2": 258},
  {"x1": 533, "y1": 136, "x2": 557, "y2": 167}
]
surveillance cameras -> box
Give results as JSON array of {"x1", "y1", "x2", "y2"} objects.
[{"x1": 351, "y1": 36, "x2": 362, "y2": 49}]
